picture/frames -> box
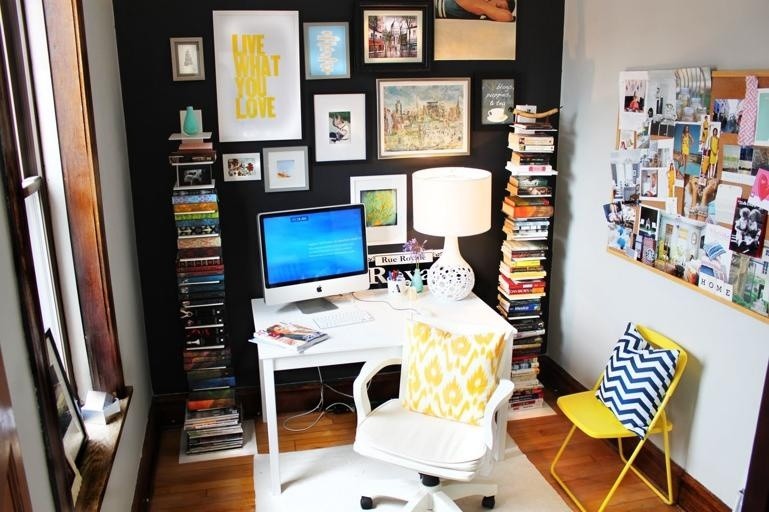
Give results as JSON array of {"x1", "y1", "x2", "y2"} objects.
[
  {"x1": 606, "y1": 68, "x2": 769, "y2": 325},
  {"x1": 60, "y1": 447, "x2": 87, "y2": 509},
  {"x1": 310, "y1": 87, "x2": 371, "y2": 166},
  {"x1": 221, "y1": 153, "x2": 261, "y2": 183},
  {"x1": 373, "y1": 76, "x2": 473, "y2": 161},
  {"x1": 476, "y1": 71, "x2": 519, "y2": 133},
  {"x1": 300, "y1": 20, "x2": 353, "y2": 82},
  {"x1": 359, "y1": 3, "x2": 431, "y2": 73},
  {"x1": 349, "y1": 174, "x2": 407, "y2": 245},
  {"x1": 211, "y1": 10, "x2": 302, "y2": 144},
  {"x1": 260, "y1": 143, "x2": 312, "y2": 194},
  {"x1": 45, "y1": 326, "x2": 90, "y2": 471},
  {"x1": 167, "y1": 36, "x2": 206, "y2": 83}
]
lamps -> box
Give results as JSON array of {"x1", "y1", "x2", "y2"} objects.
[{"x1": 410, "y1": 165, "x2": 494, "y2": 303}]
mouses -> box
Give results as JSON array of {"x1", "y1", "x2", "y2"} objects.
[{"x1": 415, "y1": 306, "x2": 435, "y2": 317}]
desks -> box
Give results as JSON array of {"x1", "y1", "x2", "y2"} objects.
[{"x1": 249, "y1": 285, "x2": 520, "y2": 496}]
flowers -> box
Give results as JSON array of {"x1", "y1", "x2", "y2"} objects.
[{"x1": 402, "y1": 237, "x2": 428, "y2": 269}]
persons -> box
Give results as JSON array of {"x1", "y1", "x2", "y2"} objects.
[
  {"x1": 329, "y1": 113, "x2": 350, "y2": 142},
  {"x1": 266, "y1": 323, "x2": 327, "y2": 342},
  {"x1": 434, "y1": 0, "x2": 516, "y2": 21},
  {"x1": 607, "y1": 171, "x2": 657, "y2": 229},
  {"x1": 620, "y1": 77, "x2": 674, "y2": 127},
  {"x1": 659, "y1": 116, "x2": 723, "y2": 220},
  {"x1": 733, "y1": 199, "x2": 760, "y2": 255}
]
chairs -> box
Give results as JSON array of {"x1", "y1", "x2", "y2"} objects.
[
  {"x1": 353, "y1": 319, "x2": 520, "y2": 511},
  {"x1": 550, "y1": 325, "x2": 688, "y2": 511}
]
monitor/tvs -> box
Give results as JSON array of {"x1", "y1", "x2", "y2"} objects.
[{"x1": 257, "y1": 203, "x2": 370, "y2": 313}]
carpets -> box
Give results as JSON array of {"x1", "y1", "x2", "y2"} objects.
[{"x1": 253, "y1": 427, "x2": 574, "y2": 512}]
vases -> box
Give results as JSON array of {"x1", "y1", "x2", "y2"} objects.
[
  {"x1": 183, "y1": 105, "x2": 202, "y2": 136},
  {"x1": 412, "y1": 269, "x2": 424, "y2": 293}
]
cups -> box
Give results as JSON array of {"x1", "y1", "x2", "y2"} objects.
[
  {"x1": 487, "y1": 108, "x2": 506, "y2": 119},
  {"x1": 387, "y1": 276, "x2": 411, "y2": 297}
]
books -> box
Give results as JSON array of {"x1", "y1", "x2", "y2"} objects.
[
  {"x1": 497, "y1": 121, "x2": 558, "y2": 416},
  {"x1": 168, "y1": 143, "x2": 214, "y2": 166},
  {"x1": 166, "y1": 184, "x2": 243, "y2": 455},
  {"x1": 251, "y1": 318, "x2": 329, "y2": 353}
]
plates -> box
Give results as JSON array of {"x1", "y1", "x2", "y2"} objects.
[{"x1": 486, "y1": 115, "x2": 508, "y2": 122}]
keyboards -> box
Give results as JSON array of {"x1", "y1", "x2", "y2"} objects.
[{"x1": 313, "y1": 309, "x2": 375, "y2": 328}]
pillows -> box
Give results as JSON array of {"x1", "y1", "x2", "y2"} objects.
[
  {"x1": 598, "y1": 321, "x2": 681, "y2": 443},
  {"x1": 400, "y1": 318, "x2": 507, "y2": 428}
]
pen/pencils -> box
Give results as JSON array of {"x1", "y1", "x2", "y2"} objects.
[{"x1": 388, "y1": 269, "x2": 399, "y2": 281}]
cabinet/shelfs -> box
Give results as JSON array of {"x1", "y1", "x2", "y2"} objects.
[
  {"x1": 497, "y1": 106, "x2": 559, "y2": 423},
  {"x1": 168, "y1": 111, "x2": 260, "y2": 465}
]
